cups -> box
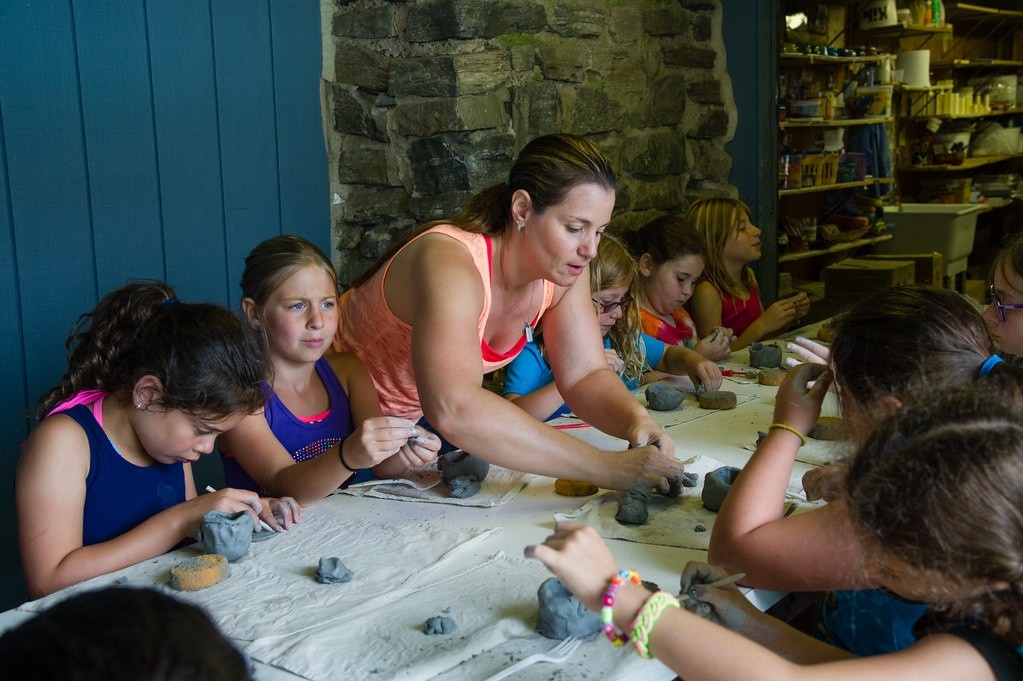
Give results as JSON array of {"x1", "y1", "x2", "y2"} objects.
[
  {"x1": 801, "y1": 226, "x2": 817, "y2": 241},
  {"x1": 788, "y1": 235, "x2": 808, "y2": 252}
]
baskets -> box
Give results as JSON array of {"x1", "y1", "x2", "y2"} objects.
[{"x1": 801, "y1": 154, "x2": 841, "y2": 185}]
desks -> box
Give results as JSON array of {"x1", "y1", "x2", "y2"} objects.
[{"x1": 0, "y1": 318, "x2": 857, "y2": 681}]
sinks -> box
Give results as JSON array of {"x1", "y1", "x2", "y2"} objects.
[{"x1": 882, "y1": 204, "x2": 988, "y2": 277}]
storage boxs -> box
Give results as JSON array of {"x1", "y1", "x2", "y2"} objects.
[
  {"x1": 863, "y1": 254, "x2": 944, "y2": 289},
  {"x1": 824, "y1": 258, "x2": 915, "y2": 302}
]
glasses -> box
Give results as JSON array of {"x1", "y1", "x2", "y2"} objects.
[
  {"x1": 592, "y1": 294, "x2": 633, "y2": 313},
  {"x1": 990, "y1": 284, "x2": 1023, "y2": 322}
]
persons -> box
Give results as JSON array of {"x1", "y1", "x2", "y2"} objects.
[
  {"x1": 14, "y1": 278, "x2": 304, "y2": 607},
  {"x1": 325, "y1": 132, "x2": 683, "y2": 499},
  {"x1": 524, "y1": 388, "x2": 1023, "y2": 681},
  {"x1": 0, "y1": 586, "x2": 254, "y2": 681},
  {"x1": 982, "y1": 237, "x2": 1023, "y2": 362},
  {"x1": 500, "y1": 232, "x2": 724, "y2": 423},
  {"x1": 218, "y1": 234, "x2": 442, "y2": 509},
  {"x1": 683, "y1": 197, "x2": 810, "y2": 352},
  {"x1": 708, "y1": 283, "x2": 1023, "y2": 658},
  {"x1": 624, "y1": 215, "x2": 737, "y2": 385}
]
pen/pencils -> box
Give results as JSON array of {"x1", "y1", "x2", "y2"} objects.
[
  {"x1": 676, "y1": 571, "x2": 745, "y2": 599},
  {"x1": 205, "y1": 485, "x2": 277, "y2": 532},
  {"x1": 553, "y1": 423, "x2": 591, "y2": 429}
]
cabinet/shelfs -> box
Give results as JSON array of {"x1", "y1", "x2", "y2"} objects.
[{"x1": 776, "y1": 0, "x2": 1023, "y2": 328}]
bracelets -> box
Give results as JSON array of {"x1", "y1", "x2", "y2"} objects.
[
  {"x1": 600, "y1": 569, "x2": 640, "y2": 648},
  {"x1": 629, "y1": 591, "x2": 680, "y2": 657},
  {"x1": 767, "y1": 423, "x2": 807, "y2": 444},
  {"x1": 501, "y1": 232, "x2": 535, "y2": 342},
  {"x1": 337, "y1": 436, "x2": 361, "y2": 472}
]
